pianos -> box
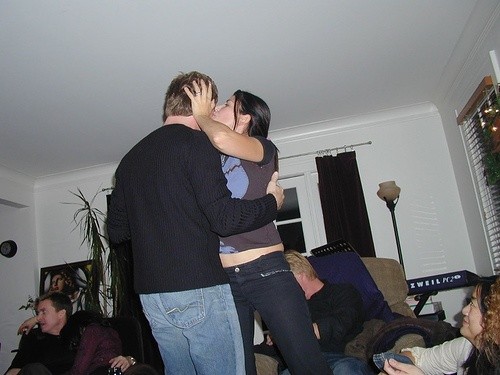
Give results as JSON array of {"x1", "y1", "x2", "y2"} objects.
[{"x1": 407, "y1": 269, "x2": 481, "y2": 294}]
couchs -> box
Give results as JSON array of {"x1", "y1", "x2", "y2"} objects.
[
  {"x1": 254, "y1": 257, "x2": 426, "y2": 375},
  {"x1": 12, "y1": 318, "x2": 158, "y2": 375}
]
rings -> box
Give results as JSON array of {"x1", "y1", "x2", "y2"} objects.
[{"x1": 196, "y1": 92, "x2": 201, "y2": 96}]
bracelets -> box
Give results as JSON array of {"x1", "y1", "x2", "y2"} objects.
[{"x1": 127, "y1": 355, "x2": 137, "y2": 366}]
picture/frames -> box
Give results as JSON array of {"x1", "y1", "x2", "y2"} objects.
[{"x1": 39, "y1": 259, "x2": 106, "y2": 317}]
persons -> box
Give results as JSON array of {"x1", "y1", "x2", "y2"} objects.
[
  {"x1": 185, "y1": 79, "x2": 334, "y2": 375},
  {"x1": 107, "y1": 71, "x2": 285, "y2": 375},
  {"x1": 48, "y1": 268, "x2": 86, "y2": 315},
  {"x1": 266, "y1": 250, "x2": 369, "y2": 375},
  {"x1": 17, "y1": 309, "x2": 123, "y2": 375},
  {"x1": 384, "y1": 275, "x2": 500, "y2": 375},
  {"x1": 4, "y1": 292, "x2": 133, "y2": 375}
]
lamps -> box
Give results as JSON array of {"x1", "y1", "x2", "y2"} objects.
[
  {"x1": 0, "y1": 240, "x2": 17, "y2": 258},
  {"x1": 376, "y1": 180, "x2": 406, "y2": 278}
]
